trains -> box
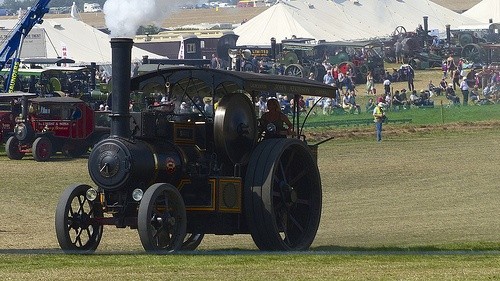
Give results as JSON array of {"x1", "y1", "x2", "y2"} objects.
[
  {"x1": 0, "y1": 54, "x2": 162, "y2": 162},
  {"x1": 54, "y1": 38, "x2": 338, "y2": 254},
  {"x1": 270, "y1": 16, "x2": 500, "y2": 82}
]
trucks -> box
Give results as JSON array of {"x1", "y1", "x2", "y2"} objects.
[{"x1": 84, "y1": 2, "x2": 102, "y2": 13}]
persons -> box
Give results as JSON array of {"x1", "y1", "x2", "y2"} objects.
[
  {"x1": 132, "y1": 63, "x2": 139, "y2": 76},
  {"x1": 366, "y1": 71, "x2": 374, "y2": 95},
  {"x1": 256, "y1": 94, "x2": 306, "y2": 114},
  {"x1": 383, "y1": 77, "x2": 391, "y2": 96},
  {"x1": 366, "y1": 88, "x2": 434, "y2": 114},
  {"x1": 372, "y1": 102, "x2": 385, "y2": 142},
  {"x1": 308, "y1": 69, "x2": 362, "y2": 116},
  {"x1": 446, "y1": 84, "x2": 460, "y2": 103},
  {"x1": 406, "y1": 65, "x2": 414, "y2": 91},
  {"x1": 429, "y1": 79, "x2": 447, "y2": 96},
  {"x1": 461, "y1": 78, "x2": 469, "y2": 106},
  {"x1": 441, "y1": 54, "x2": 463, "y2": 89},
  {"x1": 466, "y1": 66, "x2": 500, "y2": 105},
  {"x1": 394, "y1": 40, "x2": 402, "y2": 63},
  {"x1": 210, "y1": 54, "x2": 220, "y2": 69},
  {"x1": 258, "y1": 60, "x2": 284, "y2": 75},
  {"x1": 261, "y1": 98, "x2": 293, "y2": 140},
  {"x1": 95, "y1": 68, "x2": 111, "y2": 84},
  {"x1": 432, "y1": 37, "x2": 442, "y2": 47},
  {"x1": 98, "y1": 95, "x2": 222, "y2": 110}
]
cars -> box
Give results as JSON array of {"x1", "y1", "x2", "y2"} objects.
[{"x1": 185, "y1": 1, "x2": 232, "y2": 9}]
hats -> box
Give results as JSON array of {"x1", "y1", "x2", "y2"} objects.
[
  {"x1": 378, "y1": 102, "x2": 384, "y2": 108},
  {"x1": 463, "y1": 76, "x2": 467, "y2": 80},
  {"x1": 420, "y1": 89, "x2": 424, "y2": 93},
  {"x1": 351, "y1": 90, "x2": 357, "y2": 96},
  {"x1": 401, "y1": 89, "x2": 406, "y2": 92},
  {"x1": 310, "y1": 72, "x2": 314, "y2": 75},
  {"x1": 448, "y1": 83, "x2": 453, "y2": 87}
]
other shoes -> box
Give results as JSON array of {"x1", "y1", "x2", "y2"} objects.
[{"x1": 377, "y1": 139, "x2": 382, "y2": 142}]
